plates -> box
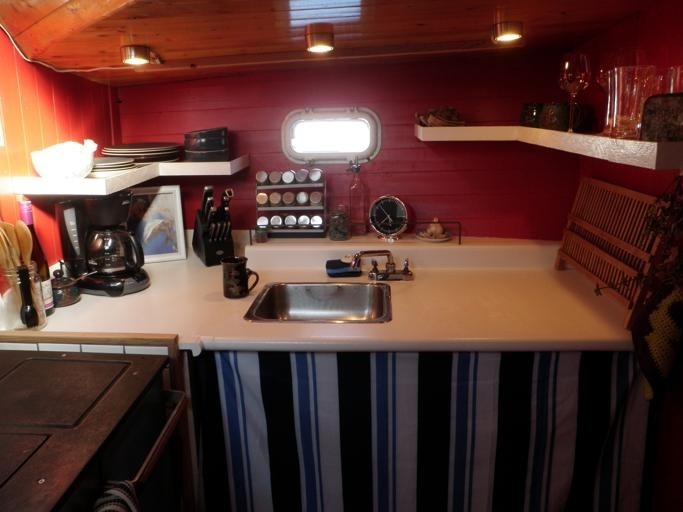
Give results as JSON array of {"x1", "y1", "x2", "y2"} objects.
[
  {"x1": 88, "y1": 142, "x2": 182, "y2": 173},
  {"x1": 415, "y1": 231, "x2": 452, "y2": 241}
]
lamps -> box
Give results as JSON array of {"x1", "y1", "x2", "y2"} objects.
[
  {"x1": 120, "y1": 44, "x2": 153, "y2": 65},
  {"x1": 490, "y1": 22, "x2": 524, "y2": 45},
  {"x1": 304, "y1": 23, "x2": 335, "y2": 54}
]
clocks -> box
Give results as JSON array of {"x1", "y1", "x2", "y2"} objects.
[{"x1": 369, "y1": 194, "x2": 409, "y2": 238}]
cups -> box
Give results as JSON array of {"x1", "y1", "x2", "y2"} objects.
[
  {"x1": 595, "y1": 63, "x2": 680, "y2": 145},
  {"x1": 0, "y1": 259, "x2": 48, "y2": 332},
  {"x1": 219, "y1": 255, "x2": 259, "y2": 300}
]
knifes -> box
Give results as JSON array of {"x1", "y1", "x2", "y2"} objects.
[{"x1": 200, "y1": 184, "x2": 235, "y2": 244}]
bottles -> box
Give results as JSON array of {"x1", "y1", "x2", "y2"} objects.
[{"x1": 18, "y1": 200, "x2": 55, "y2": 318}]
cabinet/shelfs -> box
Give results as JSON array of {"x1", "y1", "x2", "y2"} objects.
[{"x1": 255, "y1": 168, "x2": 327, "y2": 235}]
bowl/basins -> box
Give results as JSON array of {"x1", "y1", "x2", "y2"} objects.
[
  {"x1": 182, "y1": 127, "x2": 230, "y2": 163},
  {"x1": 49, "y1": 268, "x2": 82, "y2": 307}
]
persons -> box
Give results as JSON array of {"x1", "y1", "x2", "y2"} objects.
[{"x1": 128, "y1": 198, "x2": 177, "y2": 255}]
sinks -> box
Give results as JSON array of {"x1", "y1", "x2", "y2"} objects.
[{"x1": 242, "y1": 281, "x2": 393, "y2": 323}]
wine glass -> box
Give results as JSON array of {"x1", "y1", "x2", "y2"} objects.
[{"x1": 558, "y1": 51, "x2": 592, "y2": 134}]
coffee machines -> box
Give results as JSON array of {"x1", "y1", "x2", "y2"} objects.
[{"x1": 75, "y1": 188, "x2": 151, "y2": 298}]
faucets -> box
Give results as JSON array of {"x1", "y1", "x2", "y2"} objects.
[{"x1": 351, "y1": 249, "x2": 396, "y2": 274}]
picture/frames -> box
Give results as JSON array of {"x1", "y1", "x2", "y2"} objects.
[{"x1": 126, "y1": 184, "x2": 187, "y2": 261}]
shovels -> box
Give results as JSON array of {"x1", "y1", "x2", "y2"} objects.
[{"x1": 0, "y1": 219, "x2": 45, "y2": 329}]
